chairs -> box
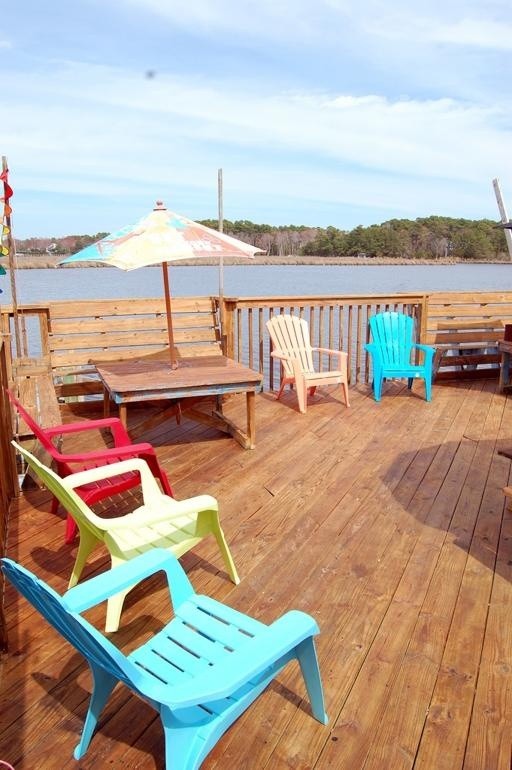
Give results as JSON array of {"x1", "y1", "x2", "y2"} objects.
[
  {"x1": 364, "y1": 312, "x2": 436, "y2": 402},
  {"x1": 6, "y1": 389, "x2": 174, "y2": 545},
  {"x1": 265, "y1": 314, "x2": 350, "y2": 414},
  {"x1": 12, "y1": 440, "x2": 240, "y2": 633},
  {"x1": 0, "y1": 548, "x2": 330, "y2": 770}
]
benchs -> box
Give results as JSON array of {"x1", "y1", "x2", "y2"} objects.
[
  {"x1": 417, "y1": 291, "x2": 512, "y2": 385},
  {"x1": 0, "y1": 310, "x2": 63, "y2": 497},
  {"x1": 47, "y1": 297, "x2": 224, "y2": 410}
]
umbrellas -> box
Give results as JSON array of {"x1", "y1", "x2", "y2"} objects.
[{"x1": 54, "y1": 201, "x2": 268, "y2": 369}]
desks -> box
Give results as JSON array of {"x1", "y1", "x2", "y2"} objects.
[
  {"x1": 95, "y1": 355, "x2": 263, "y2": 450},
  {"x1": 497, "y1": 341, "x2": 511, "y2": 395}
]
